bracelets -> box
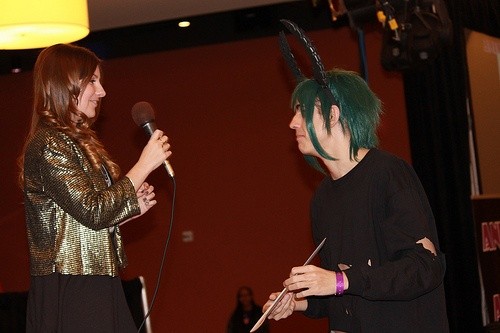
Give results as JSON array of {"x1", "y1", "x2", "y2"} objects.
[{"x1": 333, "y1": 266, "x2": 346, "y2": 298}]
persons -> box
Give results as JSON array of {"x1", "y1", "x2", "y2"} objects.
[
  {"x1": 250, "y1": 70, "x2": 450, "y2": 333},
  {"x1": 24, "y1": 44, "x2": 175, "y2": 333}
]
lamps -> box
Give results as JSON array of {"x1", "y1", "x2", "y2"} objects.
[{"x1": 0, "y1": 0, "x2": 90, "y2": 49}]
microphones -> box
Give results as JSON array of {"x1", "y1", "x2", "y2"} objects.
[{"x1": 131, "y1": 101, "x2": 175, "y2": 177}]
